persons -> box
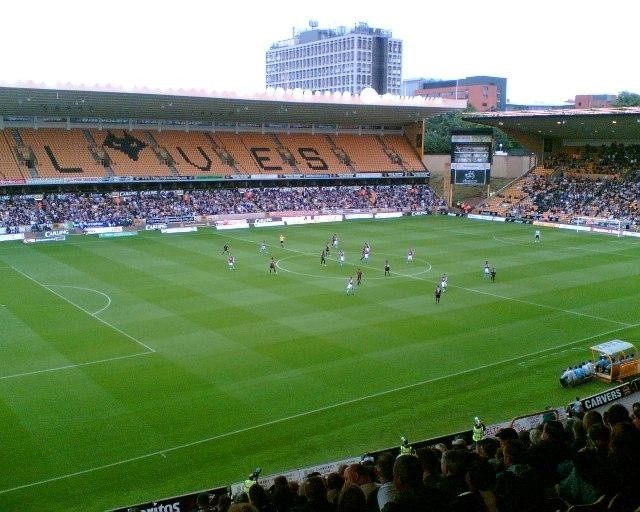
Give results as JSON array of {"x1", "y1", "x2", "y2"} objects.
[
  {"x1": 344, "y1": 276, "x2": 354, "y2": 295},
  {"x1": 441, "y1": 273, "x2": 448, "y2": 291},
  {"x1": 490, "y1": 266, "x2": 497, "y2": 282},
  {"x1": 320, "y1": 251, "x2": 327, "y2": 268},
  {"x1": 111, "y1": 350, "x2": 640, "y2": 511},
  {"x1": 2, "y1": 142, "x2": 639, "y2": 264},
  {"x1": 227, "y1": 256, "x2": 236, "y2": 270},
  {"x1": 384, "y1": 261, "x2": 391, "y2": 277},
  {"x1": 356, "y1": 268, "x2": 364, "y2": 286},
  {"x1": 268, "y1": 256, "x2": 276, "y2": 275},
  {"x1": 338, "y1": 250, "x2": 344, "y2": 266},
  {"x1": 434, "y1": 285, "x2": 441, "y2": 305},
  {"x1": 483, "y1": 261, "x2": 491, "y2": 279}
]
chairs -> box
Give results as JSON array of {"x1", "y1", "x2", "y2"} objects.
[
  {"x1": 477, "y1": 151, "x2": 639, "y2": 232},
  {"x1": 1, "y1": 124, "x2": 429, "y2": 180}
]
